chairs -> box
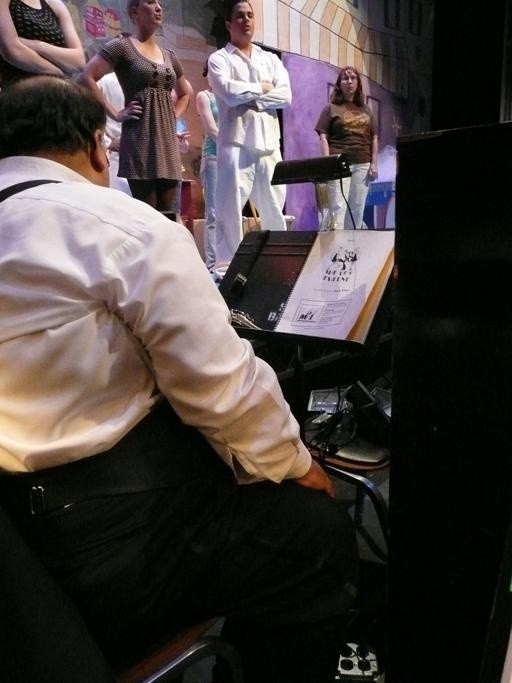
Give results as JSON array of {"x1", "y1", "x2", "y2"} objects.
[{"x1": 0, "y1": 508, "x2": 244, "y2": 683}]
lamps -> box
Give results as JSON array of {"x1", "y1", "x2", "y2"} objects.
[{"x1": 271, "y1": 154, "x2": 357, "y2": 230}]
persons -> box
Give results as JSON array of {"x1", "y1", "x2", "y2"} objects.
[
  {"x1": 0, "y1": 0, "x2": 85, "y2": 83},
  {"x1": 79, "y1": 0, "x2": 192, "y2": 211},
  {"x1": 206, "y1": 0, "x2": 293, "y2": 284},
  {"x1": 195, "y1": 59, "x2": 221, "y2": 273},
  {"x1": 92, "y1": 32, "x2": 133, "y2": 197},
  {"x1": 168, "y1": 77, "x2": 194, "y2": 223},
  {"x1": 315, "y1": 65, "x2": 381, "y2": 230},
  {"x1": 0, "y1": 71, "x2": 360, "y2": 682}
]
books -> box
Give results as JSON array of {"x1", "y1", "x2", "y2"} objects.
[{"x1": 271, "y1": 228, "x2": 396, "y2": 344}]
